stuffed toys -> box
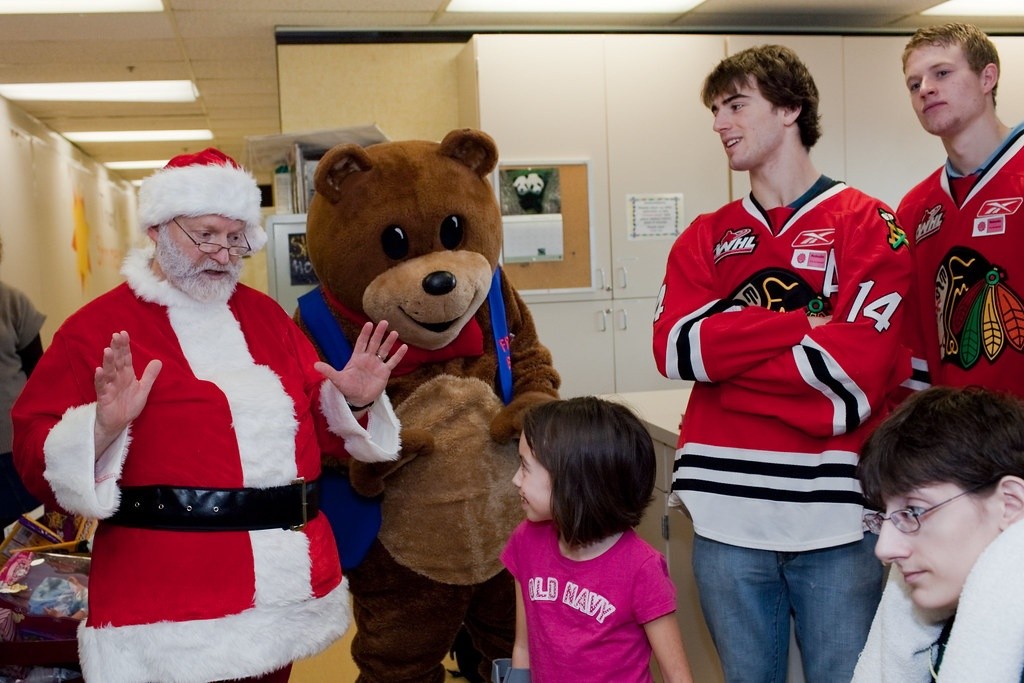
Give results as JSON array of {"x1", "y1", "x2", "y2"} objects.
[{"x1": 291, "y1": 129, "x2": 563, "y2": 683}]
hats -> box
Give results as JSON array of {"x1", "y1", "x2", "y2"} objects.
[{"x1": 136, "y1": 146, "x2": 269, "y2": 257}]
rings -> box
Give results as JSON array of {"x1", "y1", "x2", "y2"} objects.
[{"x1": 377, "y1": 353, "x2": 384, "y2": 360}]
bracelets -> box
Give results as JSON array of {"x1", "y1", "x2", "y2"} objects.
[{"x1": 347, "y1": 400, "x2": 374, "y2": 413}]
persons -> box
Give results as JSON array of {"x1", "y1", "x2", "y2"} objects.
[
  {"x1": 12, "y1": 148, "x2": 401, "y2": 683},
  {"x1": 0, "y1": 240, "x2": 46, "y2": 543},
  {"x1": 654, "y1": 45, "x2": 911, "y2": 683},
  {"x1": 894, "y1": 22, "x2": 1024, "y2": 406},
  {"x1": 497, "y1": 392, "x2": 694, "y2": 683},
  {"x1": 847, "y1": 384, "x2": 1024, "y2": 682}
]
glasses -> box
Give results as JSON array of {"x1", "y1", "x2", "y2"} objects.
[
  {"x1": 172, "y1": 218, "x2": 252, "y2": 256},
  {"x1": 862, "y1": 486, "x2": 979, "y2": 535}
]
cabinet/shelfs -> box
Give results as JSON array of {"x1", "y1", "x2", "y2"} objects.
[{"x1": 591, "y1": 390, "x2": 723, "y2": 683}]
